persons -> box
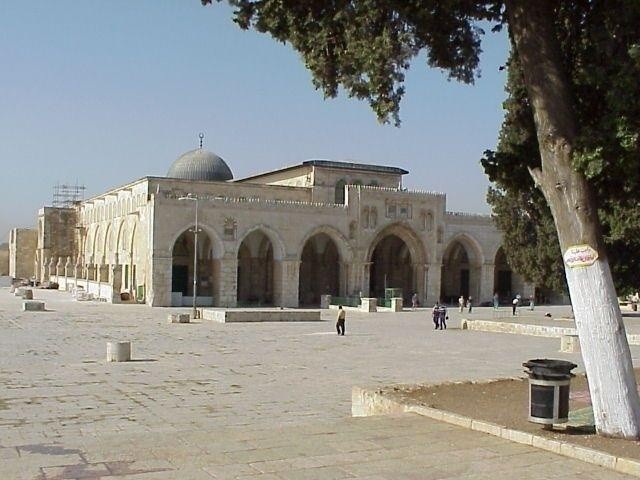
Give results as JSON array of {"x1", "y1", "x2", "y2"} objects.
[
  {"x1": 412, "y1": 293, "x2": 418, "y2": 311},
  {"x1": 439, "y1": 305, "x2": 447, "y2": 329},
  {"x1": 336, "y1": 306, "x2": 345, "y2": 335},
  {"x1": 513, "y1": 299, "x2": 518, "y2": 315},
  {"x1": 459, "y1": 295, "x2": 465, "y2": 313},
  {"x1": 432, "y1": 302, "x2": 440, "y2": 329},
  {"x1": 529, "y1": 296, "x2": 535, "y2": 310},
  {"x1": 467, "y1": 296, "x2": 473, "y2": 313}
]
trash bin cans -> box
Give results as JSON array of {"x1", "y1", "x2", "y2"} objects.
[
  {"x1": 390, "y1": 298, "x2": 403, "y2": 312},
  {"x1": 361, "y1": 297, "x2": 377, "y2": 312},
  {"x1": 522, "y1": 359, "x2": 578, "y2": 424},
  {"x1": 321, "y1": 295, "x2": 332, "y2": 309}
]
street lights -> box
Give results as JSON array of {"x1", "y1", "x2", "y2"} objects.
[{"x1": 178, "y1": 197, "x2": 202, "y2": 318}]
